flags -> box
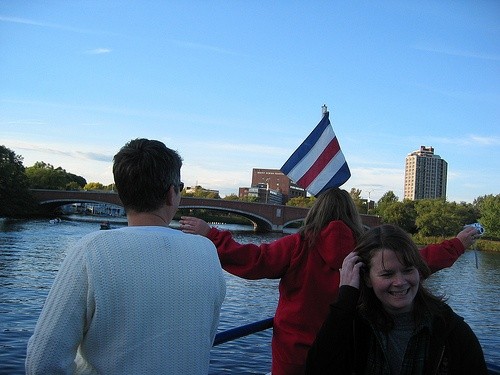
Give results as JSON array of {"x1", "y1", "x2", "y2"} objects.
[{"x1": 281, "y1": 112, "x2": 352, "y2": 199}]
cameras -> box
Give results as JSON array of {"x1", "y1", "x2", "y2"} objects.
[{"x1": 463, "y1": 223, "x2": 485, "y2": 239}]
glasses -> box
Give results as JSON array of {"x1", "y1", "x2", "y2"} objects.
[{"x1": 176, "y1": 181, "x2": 184, "y2": 192}]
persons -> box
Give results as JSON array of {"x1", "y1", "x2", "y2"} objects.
[
  {"x1": 24, "y1": 139, "x2": 227, "y2": 375},
  {"x1": 301, "y1": 224, "x2": 487, "y2": 375},
  {"x1": 178, "y1": 186, "x2": 483, "y2": 375}
]
